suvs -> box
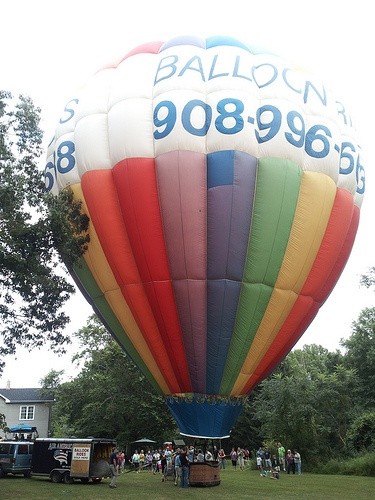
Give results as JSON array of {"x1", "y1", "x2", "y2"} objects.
[{"x1": 0, "y1": 441, "x2": 34, "y2": 478}]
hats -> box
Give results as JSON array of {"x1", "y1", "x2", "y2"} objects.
[{"x1": 287, "y1": 449, "x2": 291, "y2": 453}]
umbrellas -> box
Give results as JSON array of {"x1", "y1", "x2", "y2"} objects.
[{"x1": 130, "y1": 438, "x2": 156, "y2": 446}]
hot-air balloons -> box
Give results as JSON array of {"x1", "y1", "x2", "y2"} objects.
[{"x1": 40, "y1": 35, "x2": 370, "y2": 487}]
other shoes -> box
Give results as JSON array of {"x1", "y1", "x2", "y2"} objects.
[{"x1": 109, "y1": 484, "x2": 116, "y2": 489}]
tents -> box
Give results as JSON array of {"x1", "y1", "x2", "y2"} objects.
[{"x1": 9, "y1": 422, "x2": 36, "y2": 433}]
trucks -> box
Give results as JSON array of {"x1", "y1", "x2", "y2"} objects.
[{"x1": 32, "y1": 438, "x2": 117, "y2": 484}]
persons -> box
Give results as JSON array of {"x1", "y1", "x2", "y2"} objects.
[
  {"x1": 133, "y1": 447, "x2": 179, "y2": 474},
  {"x1": 187, "y1": 445, "x2": 213, "y2": 462},
  {"x1": 109, "y1": 447, "x2": 125, "y2": 488},
  {"x1": 217, "y1": 447, "x2": 249, "y2": 472},
  {"x1": 14, "y1": 433, "x2": 31, "y2": 440},
  {"x1": 256, "y1": 442, "x2": 301, "y2": 479},
  {"x1": 174, "y1": 447, "x2": 190, "y2": 487}
]
